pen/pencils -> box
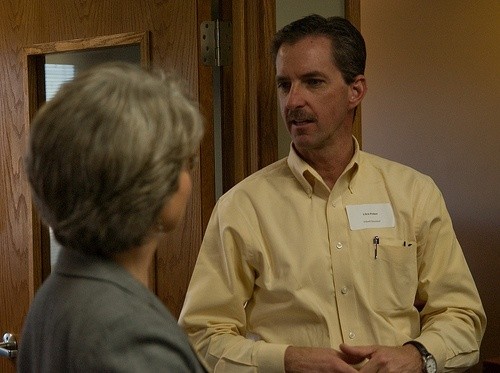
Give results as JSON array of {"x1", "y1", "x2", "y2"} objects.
[{"x1": 372, "y1": 236, "x2": 382, "y2": 261}]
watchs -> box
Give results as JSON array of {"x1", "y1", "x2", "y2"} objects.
[{"x1": 402, "y1": 340, "x2": 437, "y2": 373}]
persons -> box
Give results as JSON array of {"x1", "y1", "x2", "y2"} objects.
[
  {"x1": 178, "y1": 12, "x2": 487, "y2": 373},
  {"x1": 17, "y1": 60, "x2": 209, "y2": 373}
]
